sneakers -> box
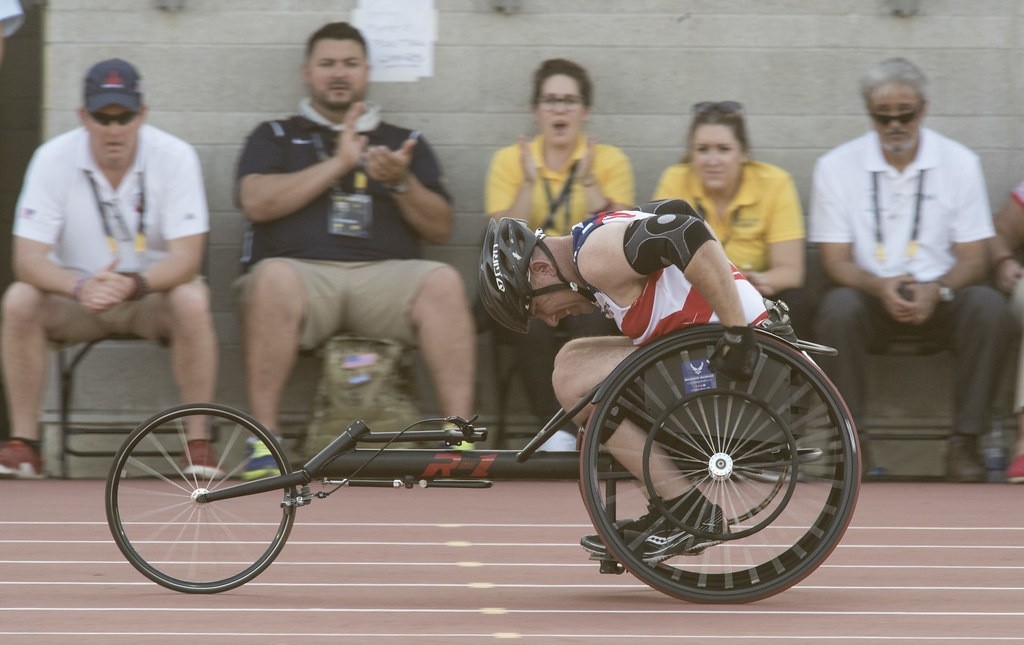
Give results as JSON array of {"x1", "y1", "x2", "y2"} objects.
[
  {"x1": 943, "y1": 434, "x2": 984, "y2": 482},
  {"x1": 439, "y1": 423, "x2": 475, "y2": 450},
  {"x1": 0, "y1": 438, "x2": 46, "y2": 479},
  {"x1": 829, "y1": 446, "x2": 872, "y2": 484},
  {"x1": 1003, "y1": 453, "x2": 1024, "y2": 484},
  {"x1": 181, "y1": 437, "x2": 226, "y2": 480},
  {"x1": 581, "y1": 503, "x2": 732, "y2": 560},
  {"x1": 240, "y1": 434, "x2": 289, "y2": 478}
]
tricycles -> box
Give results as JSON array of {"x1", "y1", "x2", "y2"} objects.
[{"x1": 105, "y1": 321, "x2": 863, "y2": 606}]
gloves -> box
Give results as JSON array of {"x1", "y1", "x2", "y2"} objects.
[{"x1": 707, "y1": 325, "x2": 762, "y2": 378}]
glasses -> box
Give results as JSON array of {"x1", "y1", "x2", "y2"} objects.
[
  {"x1": 533, "y1": 92, "x2": 585, "y2": 113},
  {"x1": 693, "y1": 101, "x2": 743, "y2": 121},
  {"x1": 867, "y1": 100, "x2": 924, "y2": 126},
  {"x1": 89, "y1": 109, "x2": 136, "y2": 127}
]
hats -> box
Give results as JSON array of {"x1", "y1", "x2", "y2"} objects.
[{"x1": 83, "y1": 57, "x2": 143, "y2": 113}]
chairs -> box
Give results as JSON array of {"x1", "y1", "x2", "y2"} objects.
[{"x1": 37, "y1": 329, "x2": 1019, "y2": 479}]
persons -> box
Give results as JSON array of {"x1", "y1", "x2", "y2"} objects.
[
  {"x1": 480, "y1": 198, "x2": 771, "y2": 559},
  {"x1": 483, "y1": 59, "x2": 638, "y2": 449},
  {"x1": 805, "y1": 59, "x2": 1024, "y2": 483},
  {"x1": 650, "y1": 102, "x2": 814, "y2": 345},
  {"x1": 0, "y1": 59, "x2": 223, "y2": 480},
  {"x1": 227, "y1": 24, "x2": 475, "y2": 479}
]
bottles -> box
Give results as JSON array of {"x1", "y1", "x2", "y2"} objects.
[{"x1": 982, "y1": 421, "x2": 1008, "y2": 483}]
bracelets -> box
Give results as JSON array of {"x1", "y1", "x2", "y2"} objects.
[
  {"x1": 120, "y1": 273, "x2": 145, "y2": 301},
  {"x1": 993, "y1": 255, "x2": 1016, "y2": 265},
  {"x1": 388, "y1": 177, "x2": 411, "y2": 193},
  {"x1": 934, "y1": 282, "x2": 955, "y2": 301},
  {"x1": 74, "y1": 276, "x2": 91, "y2": 299}
]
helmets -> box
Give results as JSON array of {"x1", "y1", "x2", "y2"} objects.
[{"x1": 478, "y1": 217, "x2": 547, "y2": 334}]
